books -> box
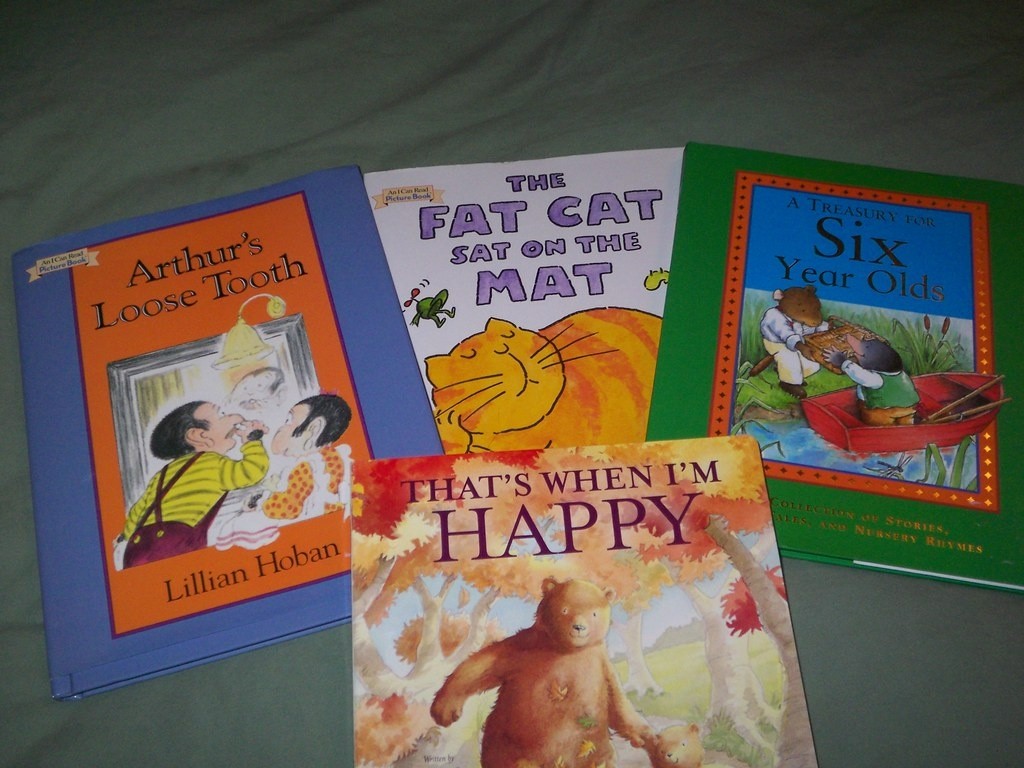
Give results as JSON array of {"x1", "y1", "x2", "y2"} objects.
[
  {"x1": 352, "y1": 436, "x2": 818, "y2": 767},
  {"x1": 15, "y1": 167, "x2": 444, "y2": 701},
  {"x1": 360, "y1": 147, "x2": 685, "y2": 454},
  {"x1": 644, "y1": 143, "x2": 1023, "y2": 594}
]
